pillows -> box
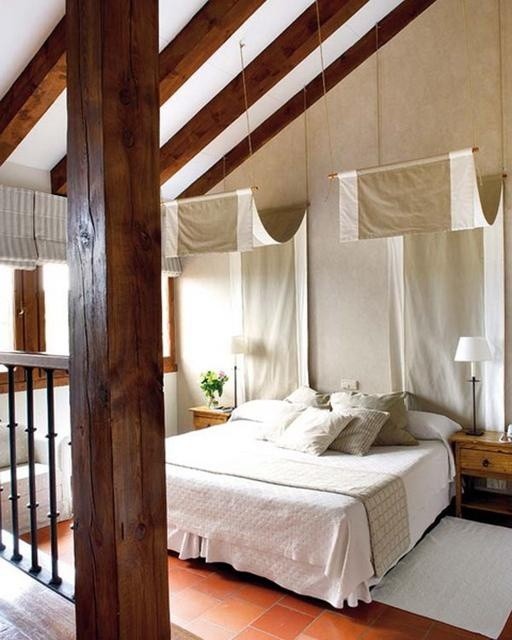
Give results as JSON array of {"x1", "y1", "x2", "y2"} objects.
[{"x1": 229, "y1": 384, "x2": 464, "y2": 458}]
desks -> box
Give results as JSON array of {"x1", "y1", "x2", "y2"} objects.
[
  {"x1": 187, "y1": 403, "x2": 231, "y2": 430},
  {"x1": 448, "y1": 426, "x2": 512, "y2": 522}
]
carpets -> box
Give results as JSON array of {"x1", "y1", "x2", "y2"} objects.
[{"x1": 368, "y1": 514, "x2": 511, "y2": 640}]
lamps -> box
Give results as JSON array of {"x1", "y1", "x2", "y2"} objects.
[
  {"x1": 453, "y1": 335, "x2": 494, "y2": 436},
  {"x1": 223, "y1": 331, "x2": 248, "y2": 414}
]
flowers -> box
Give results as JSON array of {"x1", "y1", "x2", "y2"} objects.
[{"x1": 199, "y1": 369, "x2": 227, "y2": 402}]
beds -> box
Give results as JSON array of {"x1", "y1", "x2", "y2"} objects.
[{"x1": 164, "y1": 420, "x2": 456, "y2": 610}]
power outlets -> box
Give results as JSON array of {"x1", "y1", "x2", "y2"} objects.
[{"x1": 341, "y1": 380, "x2": 359, "y2": 391}]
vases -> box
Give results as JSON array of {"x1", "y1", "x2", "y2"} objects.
[{"x1": 208, "y1": 388, "x2": 222, "y2": 408}]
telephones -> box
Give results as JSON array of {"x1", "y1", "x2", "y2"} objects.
[{"x1": 506, "y1": 422, "x2": 512, "y2": 439}]
